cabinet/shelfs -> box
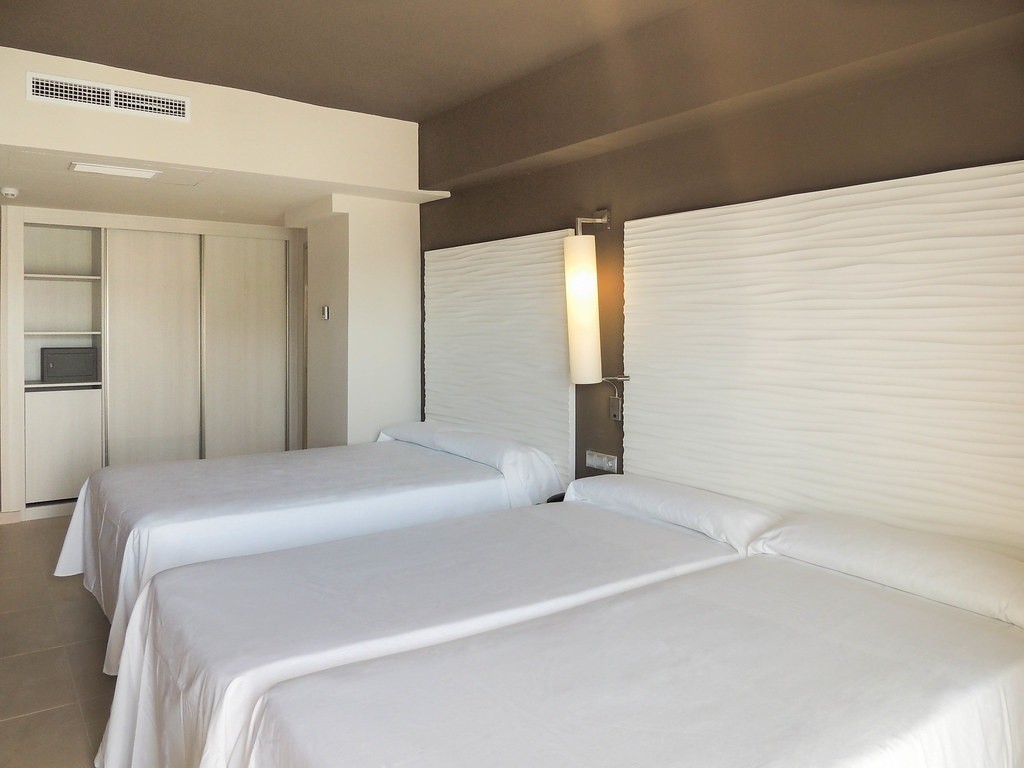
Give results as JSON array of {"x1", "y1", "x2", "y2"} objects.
[
  {"x1": 25, "y1": 390, "x2": 103, "y2": 504},
  {"x1": 24, "y1": 223, "x2": 103, "y2": 388},
  {"x1": 103, "y1": 228, "x2": 288, "y2": 468}
]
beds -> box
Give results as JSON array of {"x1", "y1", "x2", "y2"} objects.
[
  {"x1": 227, "y1": 510, "x2": 1024, "y2": 768},
  {"x1": 87, "y1": 471, "x2": 788, "y2": 768},
  {"x1": 54, "y1": 421, "x2": 566, "y2": 676}
]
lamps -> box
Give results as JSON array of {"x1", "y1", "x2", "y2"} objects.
[{"x1": 561, "y1": 208, "x2": 630, "y2": 422}]
